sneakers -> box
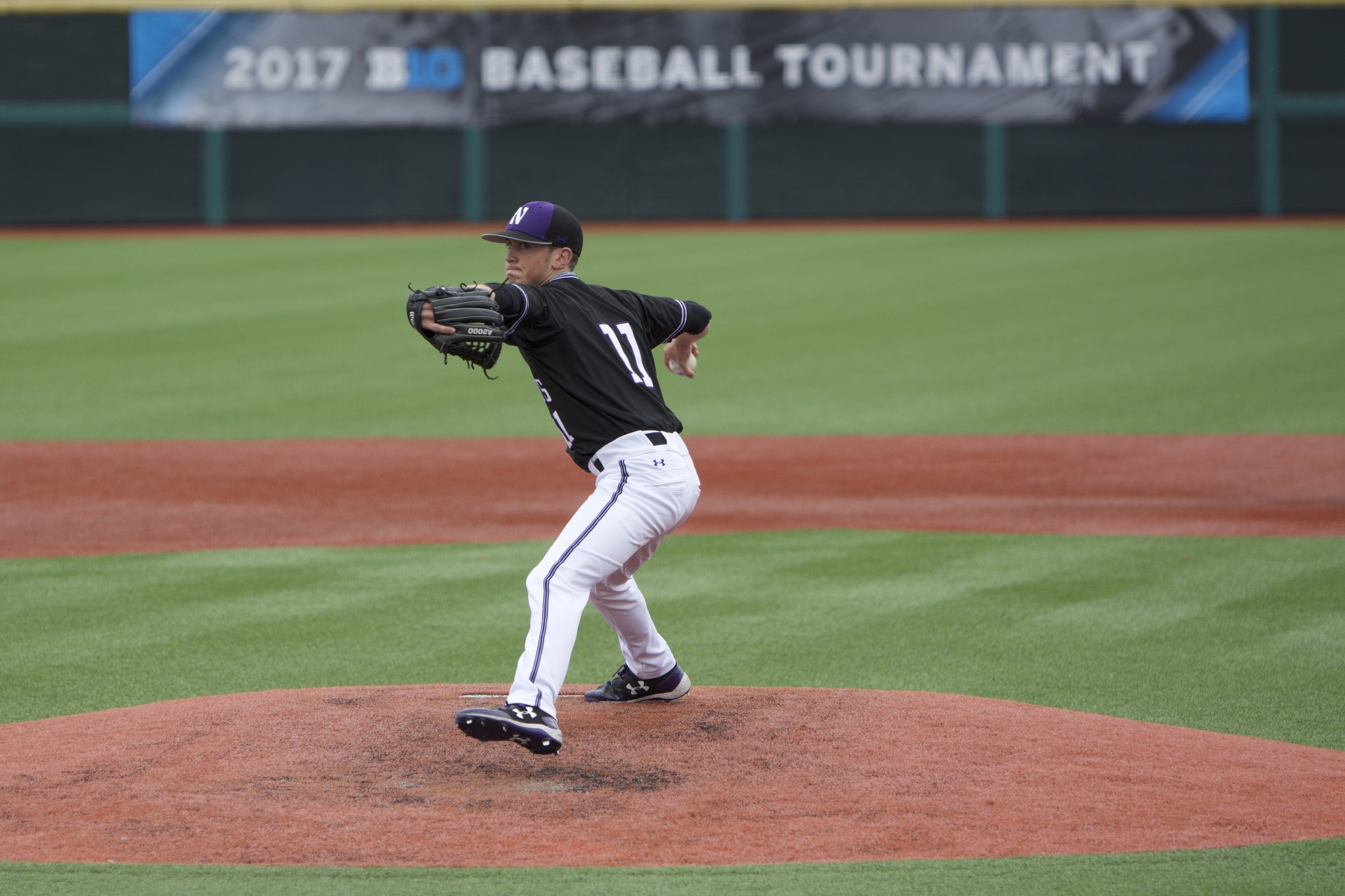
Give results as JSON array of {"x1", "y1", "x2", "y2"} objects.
[
  {"x1": 454, "y1": 703, "x2": 564, "y2": 755},
  {"x1": 584, "y1": 661, "x2": 691, "y2": 703}
]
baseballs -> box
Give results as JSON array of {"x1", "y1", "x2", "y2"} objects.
[{"x1": 668, "y1": 352, "x2": 697, "y2": 376}]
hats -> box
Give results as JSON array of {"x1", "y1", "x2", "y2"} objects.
[{"x1": 482, "y1": 201, "x2": 583, "y2": 257}]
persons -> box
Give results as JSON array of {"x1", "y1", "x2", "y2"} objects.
[{"x1": 406, "y1": 202, "x2": 714, "y2": 756}]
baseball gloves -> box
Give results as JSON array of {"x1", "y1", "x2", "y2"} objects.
[{"x1": 406, "y1": 286, "x2": 506, "y2": 369}]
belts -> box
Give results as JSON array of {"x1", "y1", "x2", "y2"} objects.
[{"x1": 593, "y1": 431, "x2": 667, "y2": 472}]
